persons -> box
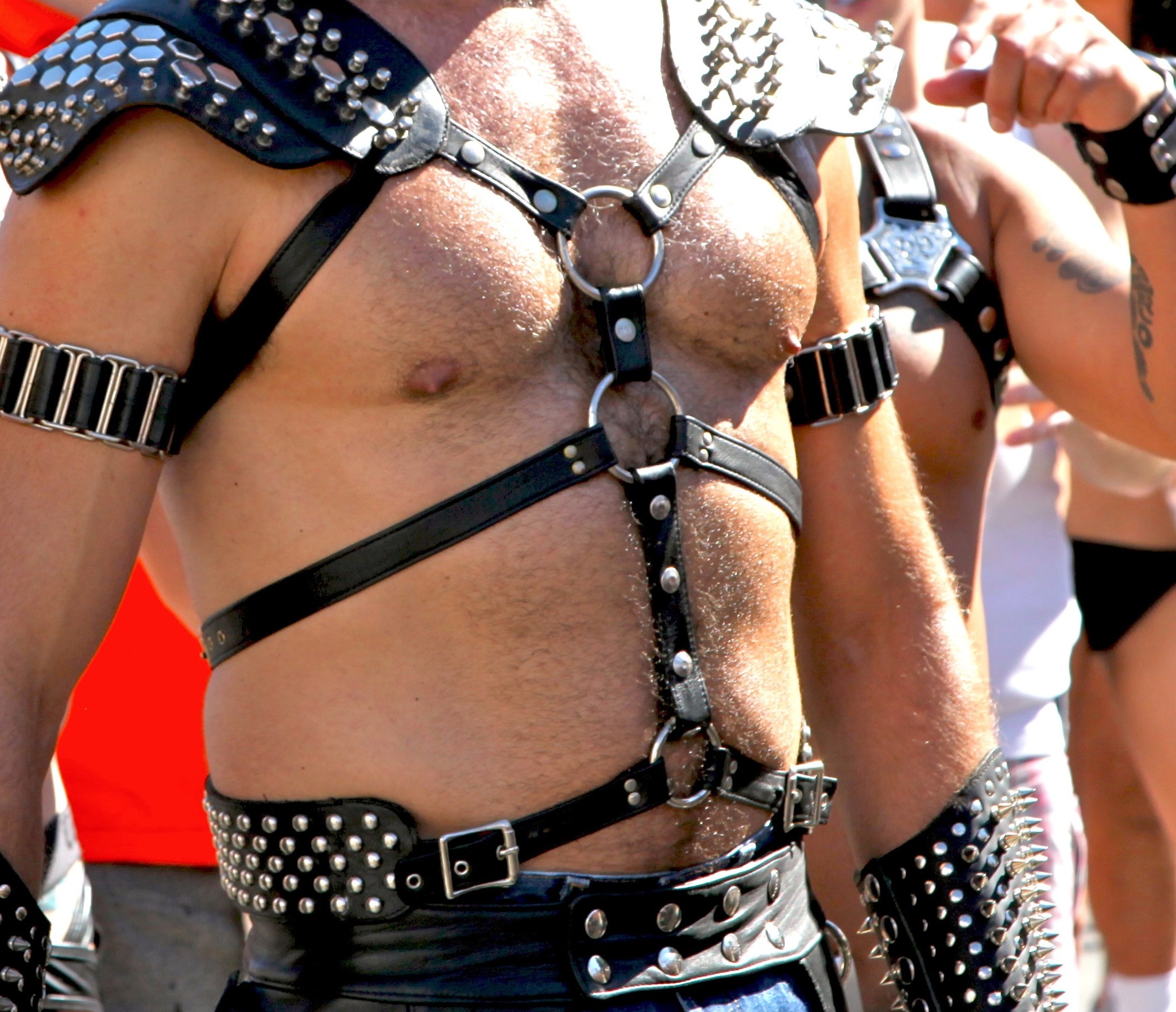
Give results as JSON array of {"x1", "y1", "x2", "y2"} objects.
[
  {"x1": 1, "y1": 0, "x2": 1176, "y2": 1010},
  {"x1": 0, "y1": 0, "x2": 1069, "y2": 1012}
]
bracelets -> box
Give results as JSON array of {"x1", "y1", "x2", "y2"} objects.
[{"x1": 1061, "y1": 62, "x2": 1176, "y2": 205}]
